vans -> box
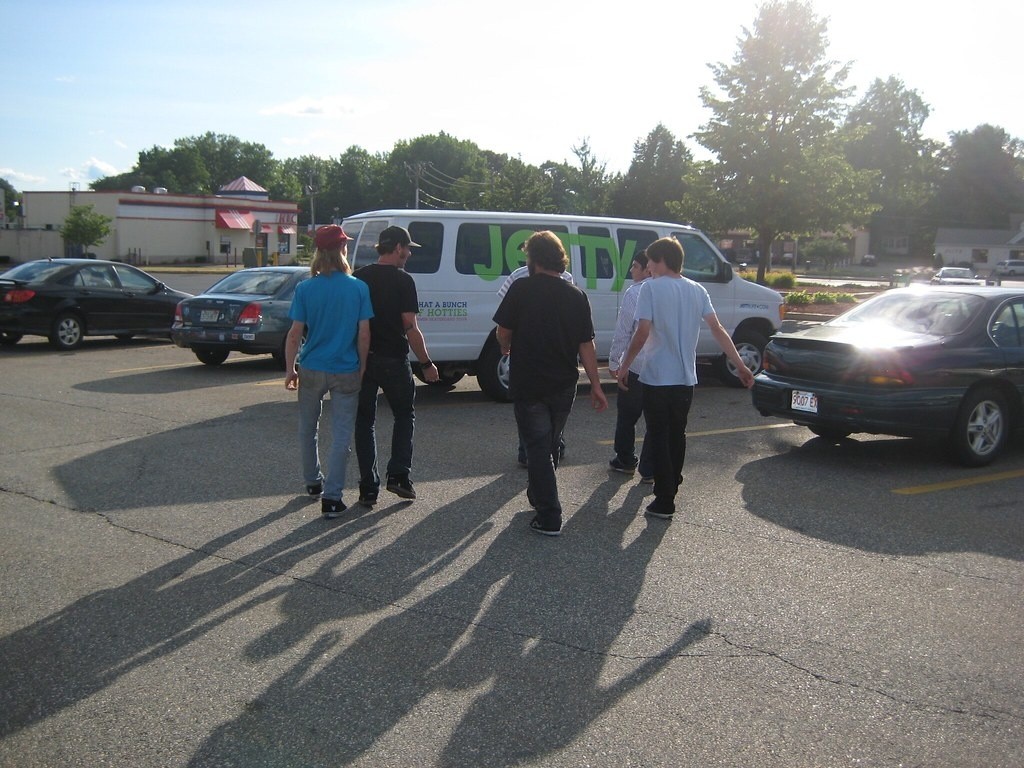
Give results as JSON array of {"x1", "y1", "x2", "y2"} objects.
[
  {"x1": 997, "y1": 259, "x2": 1024, "y2": 277},
  {"x1": 339, "y1": 208, "x2": 787, "y2": 404}
]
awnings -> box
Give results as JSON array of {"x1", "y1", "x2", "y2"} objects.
[
  {"x1": 250, "y1": 226, "x2": 272, "y2": 232},
  {"x1": 278, "y1": 226, "x2": 295, "y2": 234},
  {"x1": 216, "y1": 210, "x2": 255, "y2": 230}
]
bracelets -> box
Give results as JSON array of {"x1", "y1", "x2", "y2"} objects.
[{"x1": 420, "y1": 360, "x2": 433, "y2": 369}]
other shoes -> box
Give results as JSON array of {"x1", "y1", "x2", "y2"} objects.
[
  {"x1": 306, "y1": 481, "x2": 324, "y2": 498},
  {"x1": 386, "y1": 474, "x2": 416, "y2": 499},
  {"x1": 646, "y1": 498, "x2": 675, "y2": 518},
  {"x1": 321, "y1": 497, "x2": 348, "y2": 516},
  {"x1": 609, "y1": 457, "x2": 636, "y2": 473},
  {"x1": 530, "y1": 514, "x2": 561, "y2": 534},
  {"x1": 642, "y1": 477, "x2": 655, "y2": 483},
  {"x1": 526, "y1": 491, "x2": 536, "y2": 508},
  {"x1": 358, "y1": 492, "x2": 378, "y2": 505}
]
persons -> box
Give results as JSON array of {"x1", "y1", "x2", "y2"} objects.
[
  {"x1": 492, "y1": 231, "x2": 608, "y2": 535},
  {"x1": 285, "y1": 225, "x2": 375, "y2": 516},
  {"x1": 608, "y1": 251, "x2": 655, "y2": 483},
  {"x1": 351, "y1": 225, "x2": 438, "y2": 505},
  {"x1": 617, "y1": 236, "x2": 755, "y2": 520}
]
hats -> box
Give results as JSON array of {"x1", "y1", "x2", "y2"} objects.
[
  {"x1": 316, "y1": 226, "x2": 354, "y2": 248},
  {"x1": 379, "y1": 226, "x2": 421, "y2": 247}
]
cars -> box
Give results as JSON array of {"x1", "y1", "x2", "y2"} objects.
[
  {"x1": 930, "y1": 267, "x2": 981, "y2": 285},
  {"x1": 0, "y1": 259, "x2": 195, "y2": 353},
  {"x1": 862, "y1": 254, "x2": 877, "y2": 266},
  {"x1": 750, "y1": 283, "x2": 1024, "y2": 469},
  {"x1": 297, "y1": 244, "x2": 306, "y2": 252},
  {"x1": 170, "y1": 266, "x2": 321, "y2": 365}
]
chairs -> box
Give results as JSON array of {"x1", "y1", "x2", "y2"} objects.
[{"x1": 90, "y1": 272, "x2": 110, "y2": 287}]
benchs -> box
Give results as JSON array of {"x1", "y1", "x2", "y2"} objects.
[{"x1": 299, "y1": 258, "x2": 310, "y2": 266}]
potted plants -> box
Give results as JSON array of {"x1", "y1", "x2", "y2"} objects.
[{"x1": 6, "y1": 210, "x2": 16, "y2": 229}]
